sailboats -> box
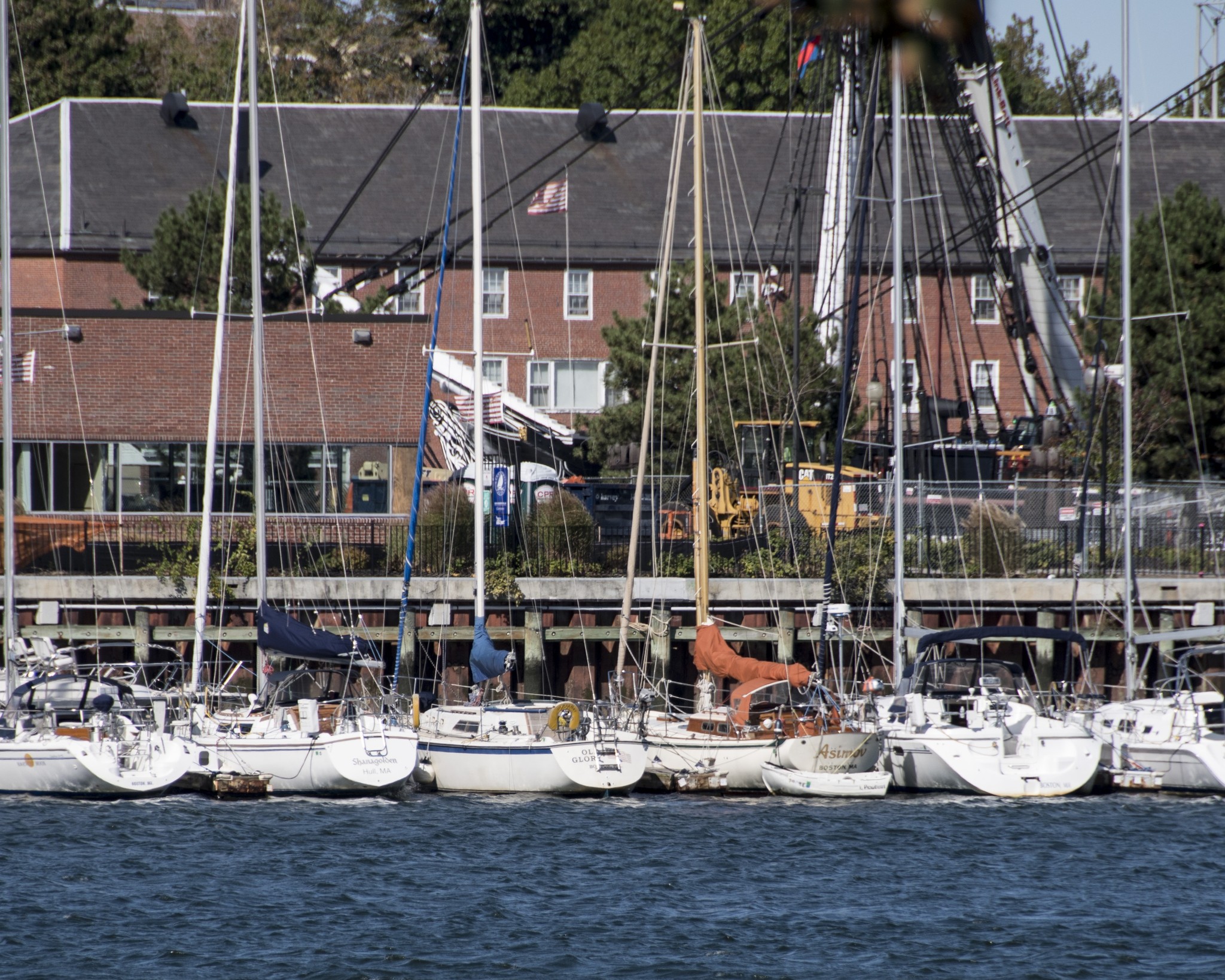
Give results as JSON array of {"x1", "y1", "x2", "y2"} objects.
[{"x1": 1, "y1": 0, "x2": 1225, "y2": 799}]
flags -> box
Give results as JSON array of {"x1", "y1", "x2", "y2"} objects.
[
  {"x1": 0, "y1": 350, "x2": 36, "y2": 383},
  {"x1": 452, "y1": 391, "x2": 504, "y2": 424},
  {"x1": 796, "y1": 32, "x2": 826, "y2": 78},
  {"x1": 526, "y1": 179, "x2": 569, "y2": 215}
]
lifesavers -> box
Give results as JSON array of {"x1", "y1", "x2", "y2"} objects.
[
  {"x1": 549, "y1": 701, "x2": 580, "y2": 731},
  {"x1": 412, "y1": 692, "x2": 420, "y2": 727}
]
